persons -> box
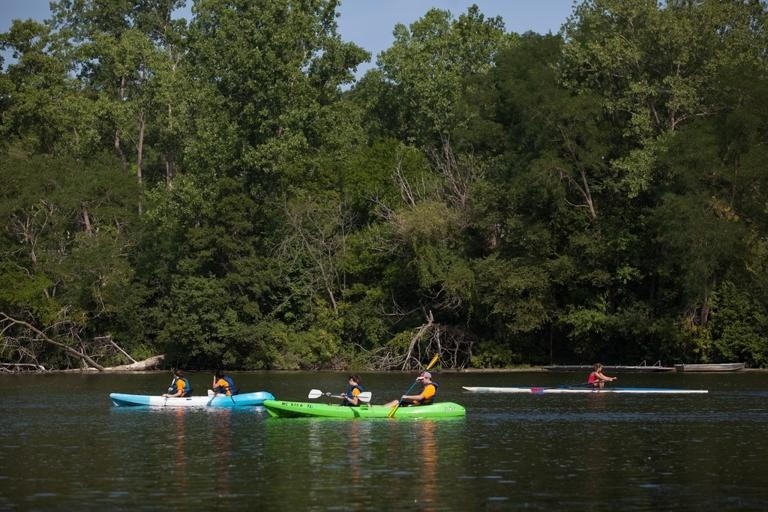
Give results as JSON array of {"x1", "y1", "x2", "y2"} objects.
[
  {"x1": 162, "y1": 370, "x2": 191, "y2": 398},
  {"x1": 384, "y1": 371, "x2": 439, "y2": 407},
  {"x1": 208, "y1": 370, "x2": 235, "y2": 396},
  {"x1": 588, "y1": 363, "x2": 617, "y2": 389},
  {"x1": 340, "y1": 374, "x2": 366, "y2": 406}
]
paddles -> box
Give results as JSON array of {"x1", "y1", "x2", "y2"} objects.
[
  {"x1": 532, "y1": 377, "x2": 618, "y2": 393},
  {"x1": 308, "y1": 388, "x2": 372, "y2": 402},
  {"x1": 387, "y1": 354, "x2": 440, "y2": 419}
]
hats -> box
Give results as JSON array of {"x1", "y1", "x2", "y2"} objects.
[{"x1": 417, "y1": 372, "x2": 432, "y2": 379}]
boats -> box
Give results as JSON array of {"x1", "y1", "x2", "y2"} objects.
[
  {"x1": 110, "y1": 389, "x2": 274, "y2": 407},
  {"x1": 673, "y1": 361, "x2": 747, "y2": 373},
  {"x1": 271, "y1": 414, "x2": 472, "y2": 420},
  {"x1": 262, "y1": 399, "x2": 467, "y2": 419},
  {"x1": 540, "y1": 359, "x2": 675, "y2": 373},
  {"x1": 462, "y1": 385, "x2": 712, "y2": 394},
  {"x1": 116, "y1": 406, "x2": 268, "y2": 413}
]
